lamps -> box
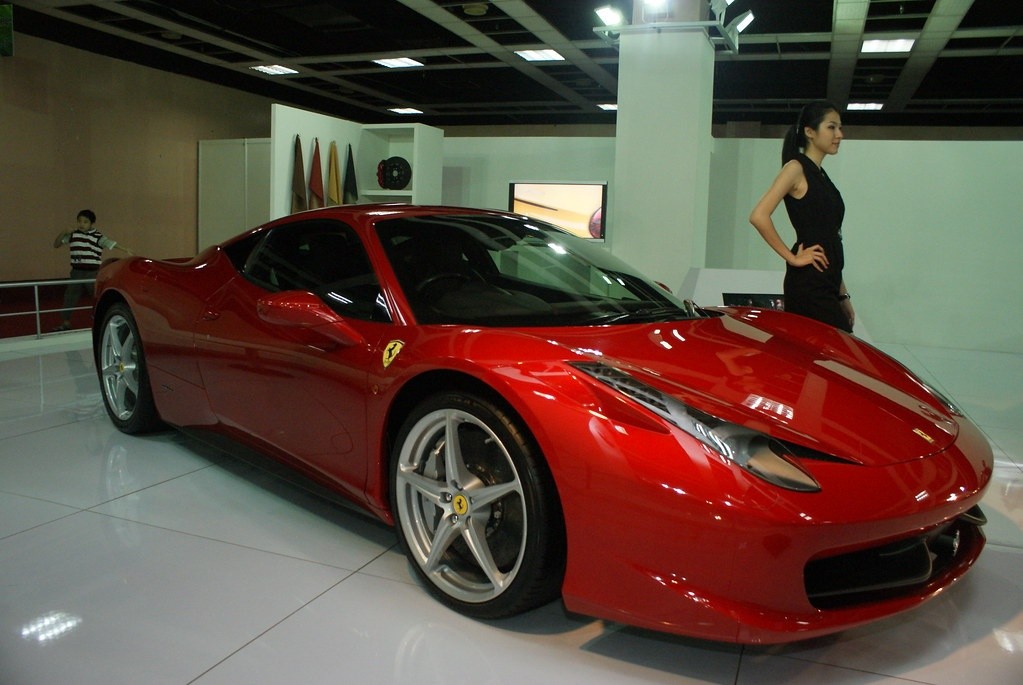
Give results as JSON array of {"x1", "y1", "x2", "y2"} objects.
[
  {"x1": 710, "y1": 0, "x2": 737, "y2": 14},
  {"x1": 643, "y1": 0, "x2": 672, "y2": 16},
  {"x1": 724, "y1": 10, "x2": 755, "y2": 39},
  {"x1": 595, "y1": 5, "x2": 629, "y2": 34}
]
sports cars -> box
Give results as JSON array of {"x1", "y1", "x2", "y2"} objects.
[{"x1": 92, "y1": 202, "x2": 994, "y2": 645}]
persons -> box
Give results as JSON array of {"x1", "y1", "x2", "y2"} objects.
[
  {"x1": 50, "y1": 210, "x2": 135, "y2": 331},
  {"x1": 749, "y1": 102, "x2": 855, "y2": 334}
]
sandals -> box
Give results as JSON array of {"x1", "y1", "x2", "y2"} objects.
[{"x1": 51, "y1": 324, "x2": 73, "y2": 332}]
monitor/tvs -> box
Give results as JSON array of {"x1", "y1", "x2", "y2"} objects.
[{"x1": 508, "y1": 179, "x2": 607, "y2": 244}]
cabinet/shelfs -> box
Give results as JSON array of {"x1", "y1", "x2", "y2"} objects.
[{"x1": 353, "y1": 124, "x2": 444, "y2": 205}]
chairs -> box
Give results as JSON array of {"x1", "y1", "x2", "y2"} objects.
[{"x1": 395, "y1": 237, "x2": 486, "y2": 277}]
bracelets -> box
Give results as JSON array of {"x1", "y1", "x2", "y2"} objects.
[{"x1": 841, "y1": 294, "x2": 850, "y2": 300}]
